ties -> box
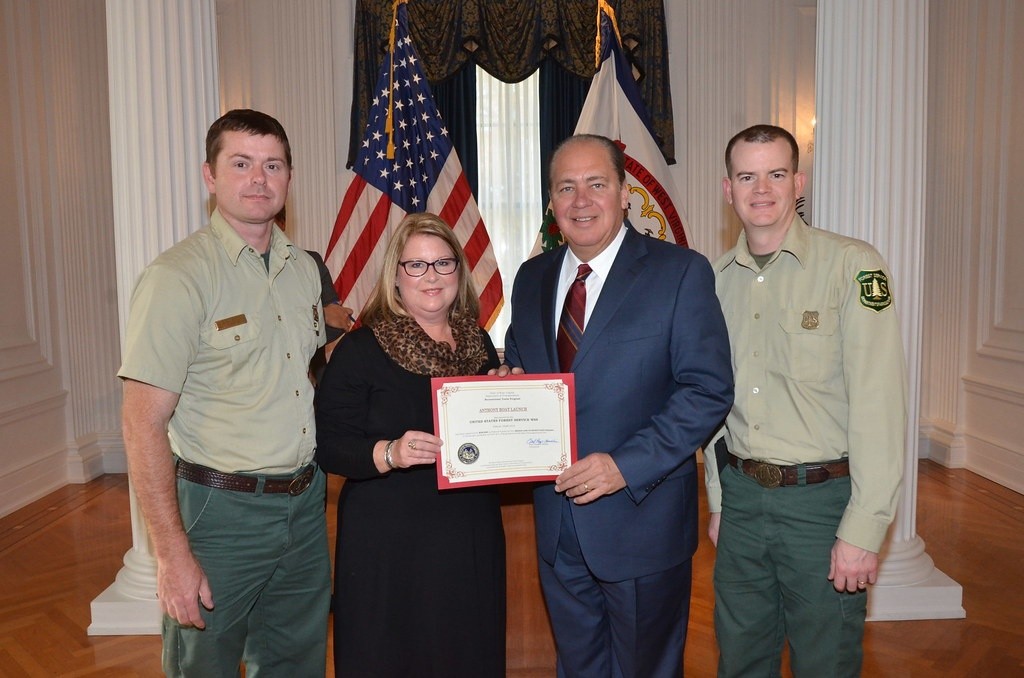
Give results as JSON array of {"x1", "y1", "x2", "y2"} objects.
[{"x1": 557, "y1": 264, "x2": 593, "y2": 373}]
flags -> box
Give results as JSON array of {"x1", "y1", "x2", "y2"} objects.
[
  {"x1": 322, "y1": 0, "x2": 503, "y2": 331},
  {"x1": 529, "y1": 0, "x2": 696, "y2": 258}
]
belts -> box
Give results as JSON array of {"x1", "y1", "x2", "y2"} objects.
[
  {"x1": 728, "y1": 454, "x2": 849, "y2": 489},
  {"x1": 175, "y1": 456, "x2": 318, "y2": 497}
]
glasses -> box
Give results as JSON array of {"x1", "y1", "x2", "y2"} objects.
[{"x1": 398, "y1": 258, "x2": 459, "y2": 277}]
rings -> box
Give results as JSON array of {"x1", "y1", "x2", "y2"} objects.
[
  {"x1": 858, "y1": 582, "x2": 867, "y2": 584},
  {"x1": 583, "y1": 483, "x2": 589, "y2": 491},
  {"x1": 348, "y1": 323, "x2": 351, "y2": 326},
  {"x1": 408, "y1": 441, "x2": 416, "y2": 448}
]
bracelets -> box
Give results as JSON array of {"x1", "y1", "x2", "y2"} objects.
[{"x1": 385, "y1": 439, "x2": 396, "y2": 469}]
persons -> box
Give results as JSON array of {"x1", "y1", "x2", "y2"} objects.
[
  {"x1": 276, "y1": 205, "x2": 354, "y2": 342},
  {"x1": 505, "y1": 135, "x2": 735, "y2": 678},
  {"x1": 313, "y1": 212, "x2": 524, "y2": 678},
  {"x1": 117, "y1": 108, "x2": 331, "y2": 678},
  {"x1": 701, "y1": 125, "x2": 903, "y2": 678}
]
